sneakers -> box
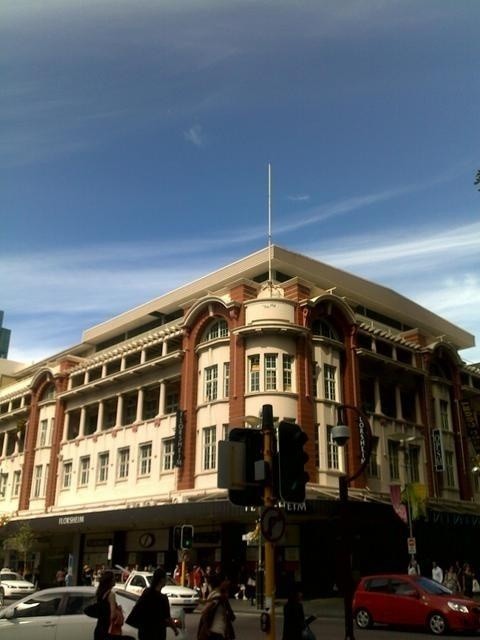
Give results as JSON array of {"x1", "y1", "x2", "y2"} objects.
[{"x1": 234, "y1": 593, "x2": 248, "y2": 601}]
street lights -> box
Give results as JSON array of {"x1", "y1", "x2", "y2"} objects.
[
  {"x1": 399, "y1": 435, "x2": 422, "y2": 576},
  {"x1": 331, "y1": 404, "x2": 373, "y2": 640}
]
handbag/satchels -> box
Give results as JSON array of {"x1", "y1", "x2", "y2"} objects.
[
  {"x1": 83, "y1": 591, "x2": 111, "y2": 619},
  {"x1": 107, "y1": 594, "x2": 125, "y2": 636},
  {"x1": 472, "y1": 579, "x2": 480, "y2": 593},
  {"x1": 301, "y1": 617, "x2": 317, "y2": 640}
]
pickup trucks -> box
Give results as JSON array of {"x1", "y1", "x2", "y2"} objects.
[{"x1": 93, "y1": 564, "x2": 200, "y2": 614}]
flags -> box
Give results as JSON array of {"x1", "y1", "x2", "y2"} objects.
[{"x1": 388, "y1": 479, "x2": 430, "y2": 527}]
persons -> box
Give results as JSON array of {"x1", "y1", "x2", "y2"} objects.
[
  {"x1": 93, "y1": 570, "x2": 123, "y2": 639},
  {"x1": 197, "y1": 572, "x2": 237, "y2": 640},
  {"x1": 137, "y1": 568, "x2": 179, "y2": 640},
  {"x1": 53, "y1": 562, "x2": 258, "y2": 601},
  {"x1": 408, "y1": 558, "x2": 480, "y2": 602},
  {"x1": 281, "y1": 588, "x2": 317, "y2": 640}
]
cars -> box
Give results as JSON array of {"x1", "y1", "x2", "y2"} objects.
[
  {"x1": 351, "y1": 573, "x2": 480, "y2": 635},
  {"x1": 0, "y1": 586, "x2": 187, "y2": 640},
  {"x1": 0, "y1": 568, "x2": 36, "y2": 603}
]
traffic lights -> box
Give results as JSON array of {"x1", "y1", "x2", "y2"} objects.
[
  {"x1": 180, "y1": 524, "x2": 194, "y2": 550},
  {"x1": 277, "y1": 421, "x2": 310, "y2": 504}
]
